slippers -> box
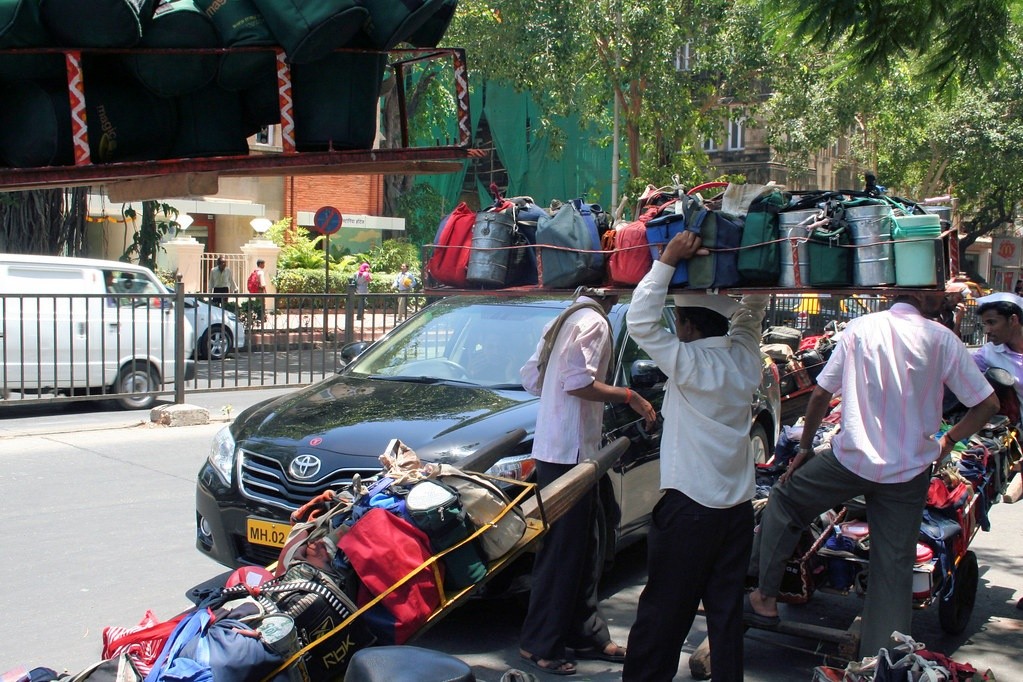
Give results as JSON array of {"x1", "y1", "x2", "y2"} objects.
[{"x1": 744, "y1": 589, "x2": 782, "y2": 629}]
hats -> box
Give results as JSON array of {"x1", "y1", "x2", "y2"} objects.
[
  {"x1": 975, "y1": 291, "x2": 1023, "y2": 311},
  {"x1": 673, "y1": 291, "x2": 741, "y2": 319}
]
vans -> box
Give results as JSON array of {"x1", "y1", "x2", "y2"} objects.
[{"x1": 0, "y1": 253, "x2": 199, "y2": 411}]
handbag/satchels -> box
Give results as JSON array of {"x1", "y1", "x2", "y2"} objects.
[
  {"x1": 427, "y1": 172, "x2": 928, "y2": 287},
  {"x1": 19, "y1": 439, "x2": 527, "y2": 682},
  {"x1": 756, "y1": 315, "x2": 1023, "y2": 682},
  {"x1": 402, "y1": 276, "x2": 413, "y2": 288}
]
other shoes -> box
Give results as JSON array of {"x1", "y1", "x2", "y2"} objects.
[
  {"x1": 357, "y1": 317, "x2": 362, "y2": 320},
  {"x1": 396, "y1": 319, "x2": 403, "y2": 323}
]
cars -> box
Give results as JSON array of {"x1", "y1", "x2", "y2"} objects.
[
  {"x1": 109, "y1": 278, "x2": 246, "y2": 361},
  {"x1": 193, "y1": 290, "x2": 782, "y2": 626}
]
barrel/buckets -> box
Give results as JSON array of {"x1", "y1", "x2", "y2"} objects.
[
  {"x1": 846, "y1": 204, "x2": 896, "y2": 286},
  {"x1": 247, "y1": 612, "x2": 311, "y2": 682},
  {"x1": 777, "y1": 208, "x2": 821, "y2": 288},
  {"x1": 466, "y1": 210, "x2": 516, "y2": 287},
  {"x1": 645, "y1": 212, "x2": 688, "y2": 287},
  {"x1": 914, "y1": 204, "x2": 951, "y2": 282},
  {"x1": 892, "y1": 214, "x2": 941, "y2": 287},
  {"x1": 913, "y1": 541, "x2": 936, "y2": 598}
]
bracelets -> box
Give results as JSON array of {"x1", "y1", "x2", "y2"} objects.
[
  {"x1": 947, "y1": 432, "x2": 957, "y2": 443},
  {"x1": 798, "y1": 448, "x2": 813, "y2": 454},
  {"x1": 624, "y1": 386, "x2": 631, "y2": 403}
]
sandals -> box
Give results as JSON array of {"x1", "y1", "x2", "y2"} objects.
[
  {"x1": 517, "y1": 647, "x2": 577, "y2": 674},
  {"x1": 565, "y1": 639, "x2": 627, "y2": 663}
]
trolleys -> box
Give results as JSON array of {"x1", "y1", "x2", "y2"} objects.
[
  {"x1": 77, "y1": 430, "x2": 631, "y2": 682},
  {"x1": 683, "y1": 359, "x2": 1023, "y2": 681}
]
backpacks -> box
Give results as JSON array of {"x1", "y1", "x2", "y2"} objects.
[{"x1": 248, "y1": 269, "x2": 261, "y2": 293}]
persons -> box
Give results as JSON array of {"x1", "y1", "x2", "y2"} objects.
[
  {"x1": 971, "y1": 292, "x2": 1023, "y2": 424},
  {"x1": 743, "y1": 290, "x2": 998, "y2": 662},
  {"x1": 391, "y1": 263, "x2": 416, "y2": 322},
  {"x1": 353, "y1": 263, "x2": 372, "y2": 319},
  {"x1": 255, "y1": 259, "x2": 268, "y2": 322},
  {"x1": 207, "y1": 257, "x2": 238, "y2": 306},
  {"x1": 623, "y1": 230, "x2": 770, "y2": 682},
  {"x1": 519, "y1": 293, "x2": 657, "y2": 675},
  {"x1": 935, "y1": 283, "x2": 967, "y2": 339}
]
